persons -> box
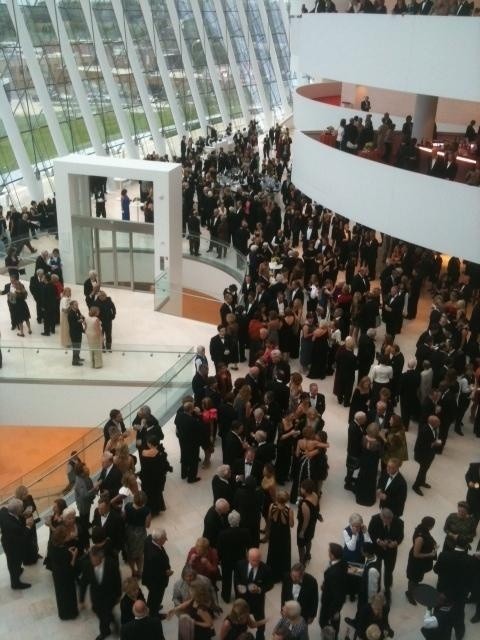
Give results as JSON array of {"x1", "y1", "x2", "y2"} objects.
[
  {"x1": 300, "y1": 1, "x2": 479, "y2": 17},
  {"x1": 42, "y1": 404, "x2": 175, "y2": 639},
  {"x1": 175, "y1": 113, "x2": 480, "y2": 640},
  {"x1": 0, "y1": 152, "x2": 174, "y2": 249},
  {"x1": 360, "y1": 95, "x2": 371, "y2": 111},
  {"x1": 0, "y1": 249, "x2": 115, "y2": 372},
  {"x1": 13, "y1": 486, "x2": 43, "y2": 567},
  {"x1": 1, "y1": 498, "x2": 32, "y2": 589}
]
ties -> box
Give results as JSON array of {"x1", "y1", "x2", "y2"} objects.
[{"x1": 248, "y1": 568, "x2": 254, "y2": 581}]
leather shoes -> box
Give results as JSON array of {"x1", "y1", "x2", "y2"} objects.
[
  {"x1": 454, "y1": 429, "x2": 464, "y2": 436},
  {"x1": 471, "y1": 616, "x2": 479, "y2": 623},
  {"x1": 406, "y1": 591, "x2": 417, "y2": 605},
  {"x1": 74, "y1": 356, "x2": 85, "y2": 365},
  {"x1": 422, "y1": 483, "x2": 431, "y2": 488},
  {"x1": 190, "y1": 250, "x2": 226, "y2": 258},
  {"x1": 412, "y1": 486, "x2": 423, "y2": 496},
  {"x1": 187, "y1": 478, "x2": 199, "y2": 483},
  {"x1": 12, "y1": 582, "x2": 30, "y2": 589},
  {"x1": 11, "y1": 319, "x2": 59, "y2": 336}
]
